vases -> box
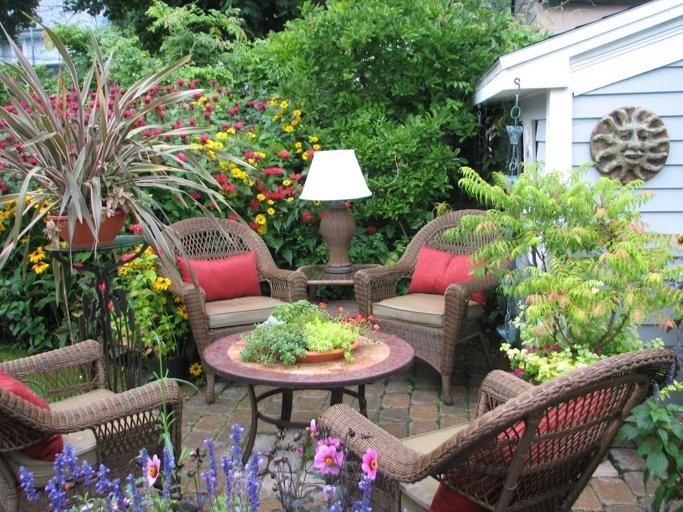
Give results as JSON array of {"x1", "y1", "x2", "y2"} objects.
[
  {"x1": 144, "y1": 350, "x2": 190, "y2": 385},
  {"x1": 256, "y1": 335, "x2": 362, "y2": 362}
]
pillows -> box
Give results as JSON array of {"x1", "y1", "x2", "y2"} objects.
[
  {"x1": 427, "y1": 385, "x2": 613, "y2": 512},
  {"x1": 175, "y1": 249, "x2": 262, "y2": 301},
  {"x1": 409, "y1": 246, "x2": 493, "y2": 306},
  {"x1": 0, "y1": 366, "x2": 64, "y2": 461}
]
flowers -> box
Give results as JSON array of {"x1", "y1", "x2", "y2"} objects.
[
  {"x1": 117, "y1": 244, "x2": 203, "y2": 376},
  {"x1": 238, "y1": 300, "x2": 380, "y2": 369}
]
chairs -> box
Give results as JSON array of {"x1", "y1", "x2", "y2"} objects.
[
  {"x1": 320, "y1": 349, "x2": 675, "y2": 512},
  {"x1": 0, "y1": 340, "x2": 183, "y2": 512},
  {"x1": 153, "y1": 216, "x2": 308, "y2": 402},
  {"x1": 352, "y1": 209, "x2": 524, "y2": 406}
]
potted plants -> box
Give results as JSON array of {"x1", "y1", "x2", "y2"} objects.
[{"x1": 0, "y1": 9, "x2": 257, "y2": 306}]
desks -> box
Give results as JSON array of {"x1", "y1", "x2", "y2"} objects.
[
  {"x1": 40, "y1": 234, "x2": 150, "y2": 397},
  {"x1": 202, "y1": 322, "x2": 408, "y2": 476}
]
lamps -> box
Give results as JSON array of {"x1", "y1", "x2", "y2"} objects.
[{"x1": 297, "y1": 149, "x2": 372, "y2": 274}]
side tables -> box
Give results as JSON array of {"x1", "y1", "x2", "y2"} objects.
[{"x1": 298, "y1": 263, "x2": 385, "y2": 314}]
